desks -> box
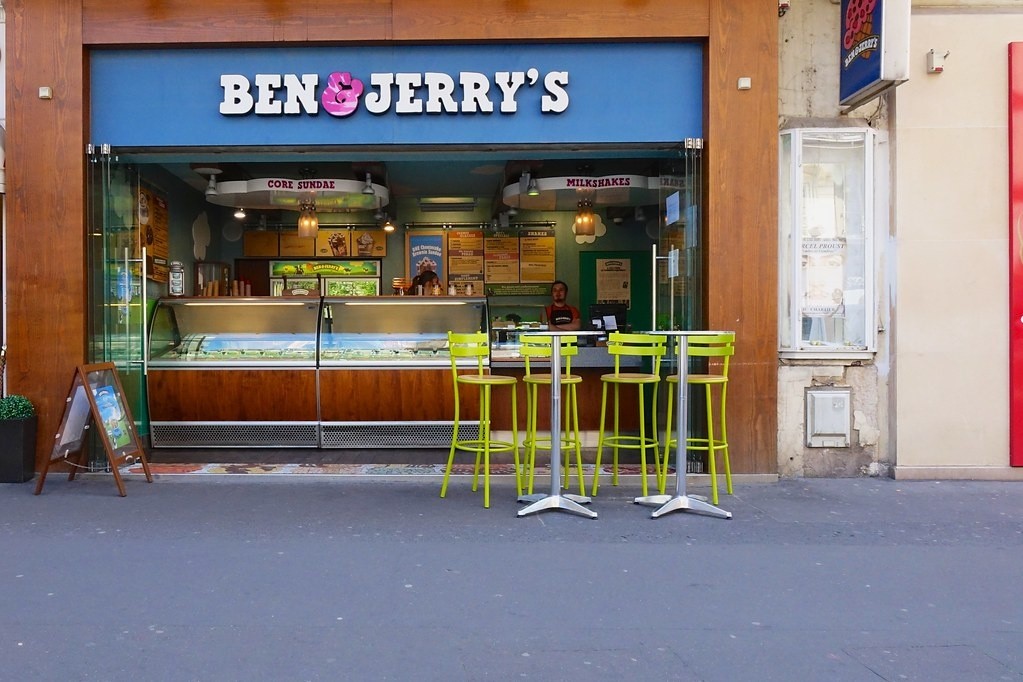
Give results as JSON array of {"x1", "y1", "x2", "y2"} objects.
[
  {"x1": 632, "y1": 330, "x2": 732, "y2": 518},
  {"x1": 518, "y1": 331, "x2": 605, "y2": 517}
]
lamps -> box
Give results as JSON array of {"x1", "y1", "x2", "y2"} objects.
[
  {"x1": 573, "y1": 200, "x2": 595, "y2": 235},
  {"x1": 362, "y1": 173, "x2": 374, "y2": 194},
  {"x1": 233, "y1": 208, "x2": 245, "y2": 218},
  {"x1": 205, "y1": 174, "x2": 217, "y2": 195},
  {"x1": 297, "y1": 202, "x2": 318, "y2": 238},
  {"x1": 383, "y1": 218, "x2": 394, "y2": 231},
  {"x1": 527, "y1": 178, "x2": 539, "y2": 196}
]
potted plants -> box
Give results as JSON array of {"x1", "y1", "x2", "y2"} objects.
[{"x1": 0, "y1": 394, "x2": 38, "y2": 483}]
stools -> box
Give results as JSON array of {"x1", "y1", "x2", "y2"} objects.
[
  {"x1": 519, "y1": 336, "x2": 585, "y2": 496},
  {"x1": 440, "y1": 330, "x2": 521, "y2": 508},
  {"x1": 591, "y1": 330, "x2": 667, "y2": 499},
  {"x1": 660, "y1": 333, "x2": 733, "y2": 505}
]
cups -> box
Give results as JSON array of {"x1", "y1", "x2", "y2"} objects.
[
  {"x1": 329, "y1": 241, "x2": 347, "y2": 256},
  {"x1": 357, "y1": 243, "x2": 373, "y2": 256}
]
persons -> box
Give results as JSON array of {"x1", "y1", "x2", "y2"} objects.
[
  {"x1": 407, "y1": 270, "x2": 441, "y2": 296},
  {"x1": 540, "y1": 281, "x2": 581, "y2": 332}
]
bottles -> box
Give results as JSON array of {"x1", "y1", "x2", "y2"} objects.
[
  {"x1": 415, "y1": 284, "x2": 426, "y2": 296},
  {"x1": 447, "y1": 284, "x2": 458, "y2": 296},
  {"x1": 431, "y1": 284, "x2": 444, "y2": 296},
  {"x1": 167, "y1": 261, "x2": 185, "y2": 297},
  {"x1": 464, "y1": 284, "x2": 474, "y2": 296}
]
